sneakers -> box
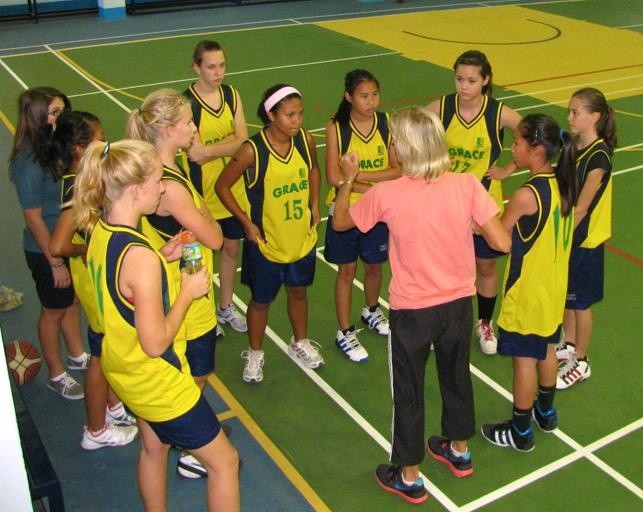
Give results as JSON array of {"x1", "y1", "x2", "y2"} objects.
[
  {"x1": 287, "y1": 333, "x2": 325, "y2": 370},
  {"x1": 427, "y1": 435, "x2": 474, "y2": 479},
  {"x1": 80, "y1": 400, "x2": 140, "y2": 451},
  {"x1": 480, "y1": 418, "x2": 536, "y2": 453},
  {"x1": 360, "y1": 303, "x2": 391, "y2": 336},
  {"x1": 46, "y1": 351, "x2": 91, "y2": 401},
  {"x1": 216, "y1": 302, "x2": 249, "y2": 338},
  {"x1": 171, "y1": 424, "x2": 244, "y2": 481},
  {"x1": 555, "y1": 350, "x2": 593, "y2": 391},
  {"x1": 241, "y1": 345, "x2": 266, "y2": 385},
  {"x1": 335, "y1": 325, "x2": 369, "y2": 363},
  {"x1": 553, "y1": 341, "x2": 577, "y2": 366},
  {"x1": 475, "y1": 318, "x2": 498, "y2": 356},
  {"x1": 531, "y1": 400, "x2": 559, "y2": 433},
  {"x1": 374, "y1": 463, "x2": 428, "y2": 505}
]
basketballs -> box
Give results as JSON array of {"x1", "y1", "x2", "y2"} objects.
[{"x1": 6, "y1": 340, "x2": 42, "y2": 386}]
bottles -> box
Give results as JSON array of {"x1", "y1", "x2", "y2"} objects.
[{"x1": 181, "y1": 233, "x2": 202, "y2": 277}]
